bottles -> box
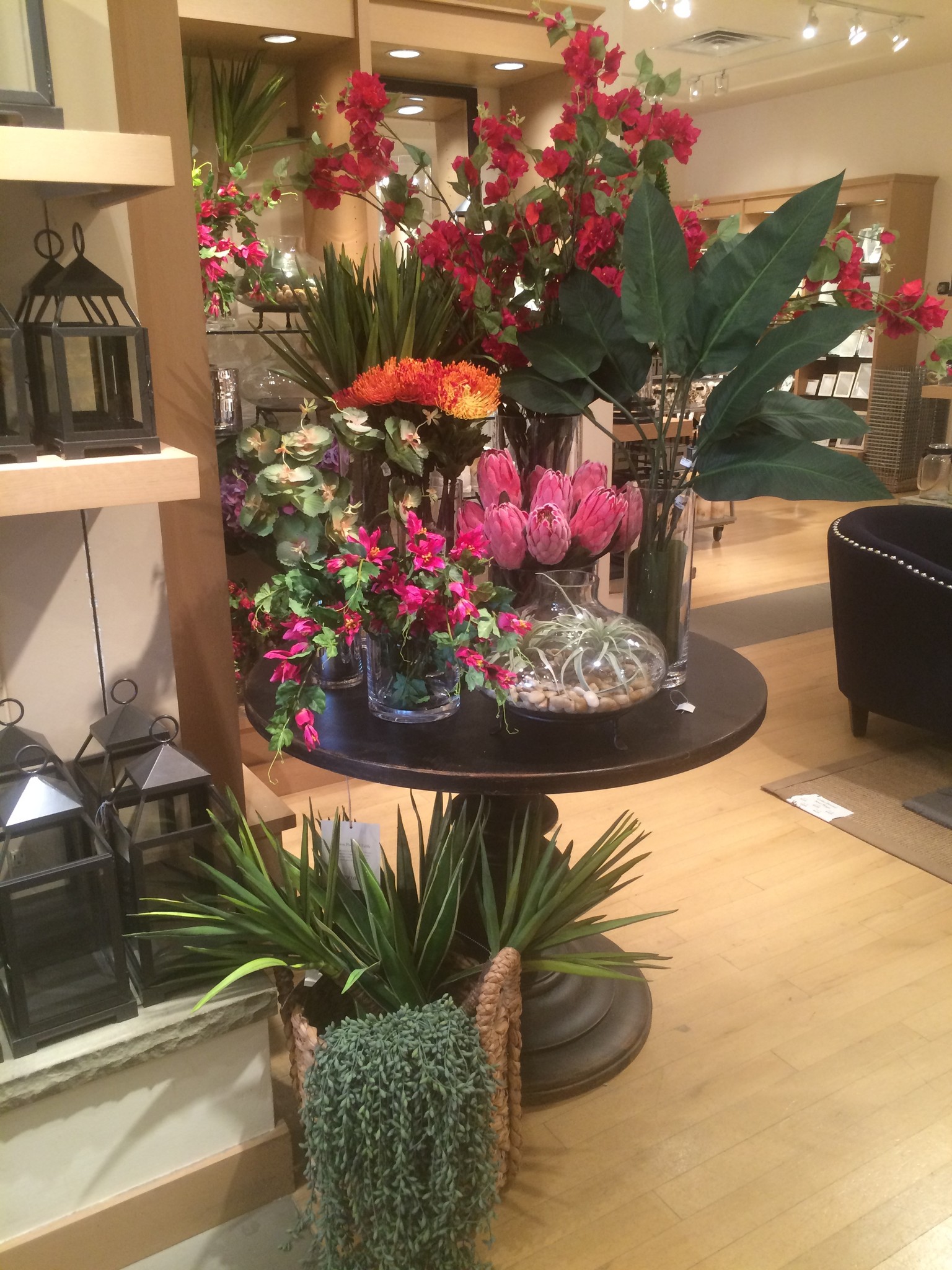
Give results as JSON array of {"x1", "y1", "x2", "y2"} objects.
[
  {"x1": 236, "y1": 336, "x2": 337, "y2": 409},
  {"x1": 919, "y1": 443, "x2": 952, "y2": 500}
]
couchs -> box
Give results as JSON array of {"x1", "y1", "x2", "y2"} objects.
[{"x1": 827, "y1": 505, "x2": 952, "y2": 737}]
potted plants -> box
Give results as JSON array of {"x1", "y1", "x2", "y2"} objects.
[{"x1": 123, "y1": 784, "x2": 680, "y2": 1270}]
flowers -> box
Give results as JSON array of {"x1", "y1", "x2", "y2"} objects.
[{"x1": 182, "y1": 5, "x2": 952, "y2": 764}]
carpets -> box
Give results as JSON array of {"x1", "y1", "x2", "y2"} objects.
[{"x1": 764, "y1": 739, "x2": 952, "y2": 883}]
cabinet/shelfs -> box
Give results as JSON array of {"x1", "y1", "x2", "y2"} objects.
[
  {"x1": 899, "y1": 383, "x2": 952, "y2": 508},
  {"x1": 641, "y1": 352, "x2": 725, "y2": 473},
  {"x1": 605, "y1": 418, "x2": 736, "y2": 542},
  {"x1": 780, "y1": 354, "x2": 876, "y2": 477},
  {"x1": 0, "y1": 123, "x2": 276, "y2": 1111}
]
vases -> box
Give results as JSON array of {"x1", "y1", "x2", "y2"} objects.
[
  {"x1": 366, "y1": 626, "x2": 461, "y2": 722},
  {"x1": 402, "y1": 480, "x2": 463, "y2": 563},
  {"x1": 307, "y1": 597, "x2": 364, "y2": 690},
  {"x1": 202, "y1": 273, "x2": 237, "y2": 329}
]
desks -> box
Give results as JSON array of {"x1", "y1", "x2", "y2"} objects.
[{"x1": 239, "y1": 615, "x2": 768, "y2": 1102}]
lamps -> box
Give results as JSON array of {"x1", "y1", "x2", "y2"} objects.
[
  {"x1": 884, "y1": 16, "x2": 908, "y2": 52},
  {"x1": 687, "y1": 72, "x2": 703, "y2": 101},
  {"x1": 846, "y1": 9, "x2": 867, "y2": 45},
  {"x1": 803, "y1": 6, "x2": 819, "y2": 39},
  {"x1": 713, "y1": 69, "x2": 729, "y2": 96}
]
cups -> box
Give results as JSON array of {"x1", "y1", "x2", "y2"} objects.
[{"x1": 209, "y1": 365, "x2": 244, "y2": 437}]
